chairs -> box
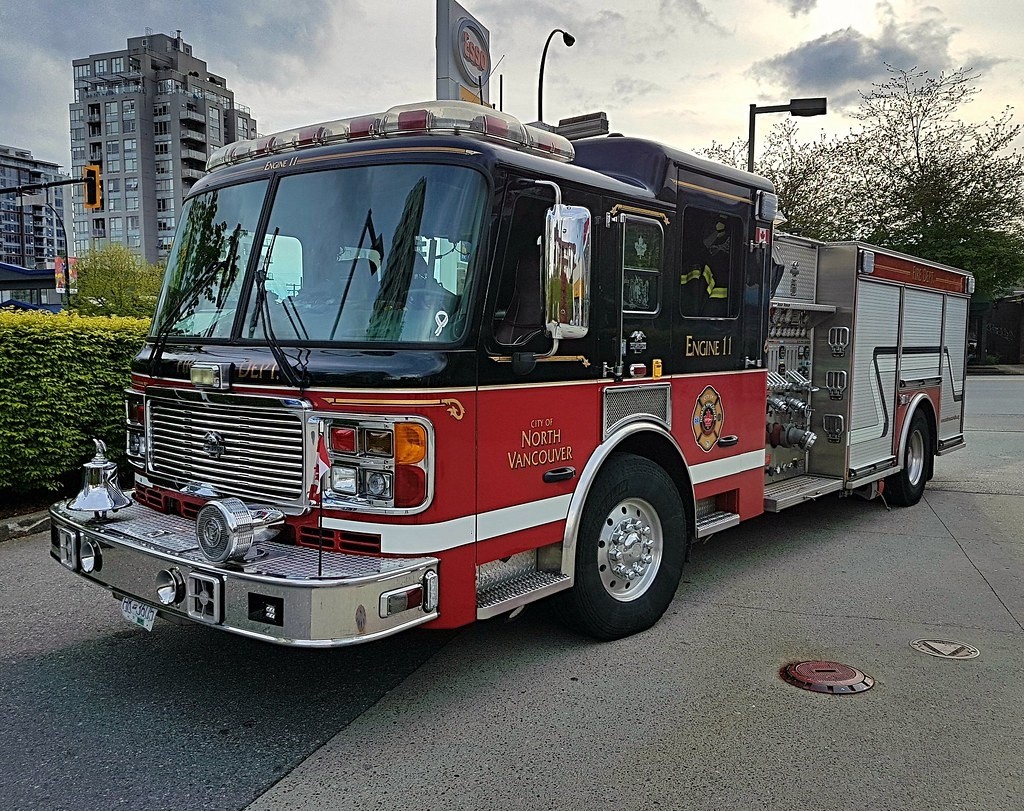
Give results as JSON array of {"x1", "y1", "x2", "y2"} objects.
[
  {"x1": 497, "y1": 242, "x2": 543, "y2": 344},
  {"x1": 410, "y1": 252, "x2": 437, "y2": 290},
  {"x1": 331, "y1": 258, "x2": 377, "y2": 301}
]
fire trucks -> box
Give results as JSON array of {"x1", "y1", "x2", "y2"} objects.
[{"x1": 37, "y1": 93, "x2": 976, "y2": 657}]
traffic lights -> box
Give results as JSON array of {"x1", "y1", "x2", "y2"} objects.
[{"x1": 83, "y1": 165, "x2": 103, "y2": 209}]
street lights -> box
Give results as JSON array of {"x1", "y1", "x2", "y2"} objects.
[
  {"x1": 747, "y1": 94, "x2": 830, "y2": 175},
  {"x1": 536, "y1": 26, "x2": 575, "y2": 124}
]
